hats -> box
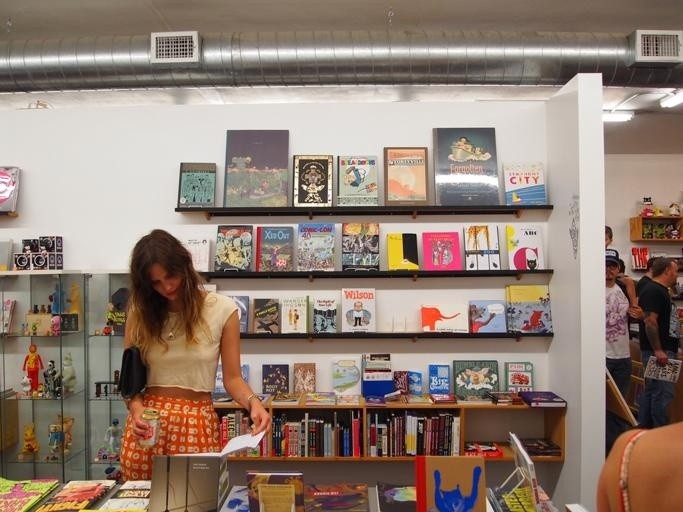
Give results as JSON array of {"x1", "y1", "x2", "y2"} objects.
[{"x1": 605, "y1": 248, "x2": 619, "y2": 264}]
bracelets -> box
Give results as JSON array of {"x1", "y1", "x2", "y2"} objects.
[
  {"x1": 245, "y1": 394, "x2": 262, "y2": 413},
  {"x1": 617, "y1": 430, "x2": 647, "y2": 511}
]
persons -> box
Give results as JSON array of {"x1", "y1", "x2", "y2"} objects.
[
  {"x1": 98, "y1": 418, "x2": 122, "y2": 460},
  {"x1": 605, "y1": 225, "x2": 614, "y2": 249},
  {"x1": 22, "y1": 345, "x2": 44, "y2": 391},
  {"x1": 636, "y1": 259, "x2": 652, "y2": 359},
  {"x1": 58, "y1": 416, "x2": 75, "y2": 456},
  {"x1": 606, "y1": 250, "x2": 645, "y2": 400},
  {"x1": 20, "y1": 376, "x2": 33, "y2": 398},
  {"x1": 42, "y1": 360, "x2": 64, "y2": 400},
  {"x1": 596, "y1": 422, "x2": 683, "y2": 512},
  {"x1": 117, "y1": 229, "x2": 272, "y2": 480},
  {"x1": 639, "y1": 258, "x2": 680, "y2": 428},
  {"x1": 45, "y1": 424, "x2": 64, "y2": 461},
  {"x1": 352, "y1": 302, "x2": 365, "y2": 327},
  {"x1": 19, "y1": 278, "x2": 81, "y2": 335},
  {"x1": 95, "y1": 302, "x2": 117, "y2": 336},
  {"x1": 616, "y1": 259, "x2": 630, "y2": 303},
  {"x1": 54, "y1": 352, "x2": 78, "y2": 392}
]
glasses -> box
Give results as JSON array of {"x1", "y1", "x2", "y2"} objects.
[{"x1": 606, "y1": 263, "x2": 618, "y2": 269}]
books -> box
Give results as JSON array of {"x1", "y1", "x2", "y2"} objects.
[
  {"x1": 341, "y1": 222, "x2": 381, "y2": 273},
  {"x1": 337, "y1": 155, "x2": 379, "y2": 206},
  {"x1": 431, "y1": 394, "x2": 456, "y2": 404},
  {"x1": 292, "y1": 154, "x2": 333, "y2": 207},
  {"x1": 407, "y1": 371, "x2": 424, "y2": 395},
  {"x1": 253, "y1": 298, "x2": 280, "y2": 333},
  {"x1": 271, "y1": 393, "x2": 302, "y2": 406},
  {"x1": 362, "y1": 353, "x2": 395, "y2": 394},
  {"x1": 418, "y1": 303, "x2": 469, "y2": 333},
  {"x1": 463, "y1": 225, "x2": 501, "y2": 271},
  {"x1": 521, "y1": 437, "x2": 562, "y2": 457},
  {"x1": 213, "y1": 364, "x2": 250, "y2": 401},
  {"x1": 365, "y1": 394, "x2": 386, "y2": 406},
  {"x1": 262, "y1": 364, "x2": 290, "y2": 395},
  {"x1": 0, "y1": 240, "x2": 14, "y2": 270},
  {"x1": 98, "y1": 479, "x2": 151, "y2": 511},
  {"x1": 631, "y1": 247, "x2": 649, "y2": 270},
  {"x1": 294, "y1": 362, "x2": 316, "y2": 394},
  {"x1": 0, "y1": 298, "x2": 18, "y2": 335},
  {"x1": 220, "y1": 486, "x2": 248, "y2": 512},
  {"x1": 505, "y1": 284, "x2": 554, "y2": 333},
  {"x1": 273, "y1": 410, "x2": 364, "y2": 457},
  {"x1": 487, "y1": 392, "x2": 524, "y2": 406},
  {"x1": 415, "y1": 458, "x2": 487, "y2": 512},
  {"x1": 306, "y1": 481, "x2": 369, "y2": 511},
  {"x1": 297, "y1": 223, "x2": 335, "y2": 271},
  {"x1": 644, "y1": 355, "x2": 682, "y2": 383},
  {"x1": 464, "y1": 441, "x2": 504, "y2": 458},
  {"x1": 35, "y1": 480, "x2": 116, "y2": 512},
  {"x1": 258, "y1": 484, "x2": 296, "y2": 512},
  {"x1": 504, "y1": 161, "x2": 548, "y2": 206},
  {"x1": 196, "y1": 283, "x2": 218, "y2": 292},
  {"x1": 432, "y1": 126, "x2": 501, "y2": 207},
  {"x1": 453, "y1": 360, "x2": 500, "y2": 400},
  {"x1": 468, "y1": 299, "x2": 506, "y2": 333},
  {"x1": 428, "y1": 365, "x2": 450, "y2": 394},
  {"x1": 305, "y1": 392, "x2": 335, "y2": 406},
  {"x1": 367, "y1": 413, "x2": 461, "y2": 458},
  {"x1": 179, "y1": 237, "x2": 210, "y2": 273},
  {"x1": 341, "y1": 288, "x2": 376, "y2": 332},
  {"x1": 422, "y1": 233, "x2": 461, "y2": 271},
  {"x1": 518, "y1": 392, "x2": 567, "y2": 408},
  {"x1": 506, "y1": 223, "x2": 545, "y2": 270},
  {"x1": 223, "y1": 130, "x2": 289, "y2": 207},
  {"x1": 377, "y1": 481, "x2": 417, "y2": 512},
  {"x1": 224, "y1": 296, "x2": 249, "y2": 333},
  {"x1": 218, "y1": 410, "x2": 268, "y2": 458},
  {"x1": 178, "y1": 162, "x2": 217, "y2": 209},
  {"x1": 387, "y1": 233, "x2": 418, "y2": 270},
  {"x1": 385, "y1": 146, "x2": 429, "y2": 207},
  {"x1": 215, "y1": 225, "x2": 254, "y2": 272},
  {"x1": 394, "y1": 371, "x2": 408, "y2": 394},
  {"x1": 247, "y1": 470, "x2": 305, "y2": 511},
  {"x1": 0, "y1": 478, "x2": 60, "y2": 512},
  {"x1": 509, "y1": 431, "x2": 542, "y2": 511},
  {"x1": 313, "y1": 297, "x2": 337, "y2": 334},
  {"x1": 405, "y1": 394, "x2": 430, "y2": 404},
  {"x1": 281, "y1": 297, "x2": 309, "y2": 332},
  {"x1": 330, "y1": 358, "x2": 362, "y2": 395},
  {"x1": 257, "y1": 227, "x2": 294, "y2": 274},
  {"x1": 504, "y1": 361, "x2": 534, "y2": 392},
  {"x1": 337, "y1": 396, "x2": 360, "y2": 406}
]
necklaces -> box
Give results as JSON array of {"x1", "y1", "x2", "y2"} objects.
[{"x1": 160, "y1": 322, "x2": 185, "y2": 341}]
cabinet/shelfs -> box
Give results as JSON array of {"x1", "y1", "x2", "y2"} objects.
[
  {"x1": 629, "y1": 215, "x2": 683, "y2": 243},
  {"x1": 0, "y1": 272, "x2": 89, "y2": 480},
  {"x1": 89, "y1": 270, "x2": 133, "y2": 473},
  {"x1": 199, "y1": 203, "x2": 555, "y2": 406},
  {"x1": 211, "y1": 395, "x2": 567, "y2": 464}
]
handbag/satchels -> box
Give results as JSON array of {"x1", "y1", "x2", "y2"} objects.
[{"x1": 115, "y1": 348, "x2": 146, "y2": 399}]
